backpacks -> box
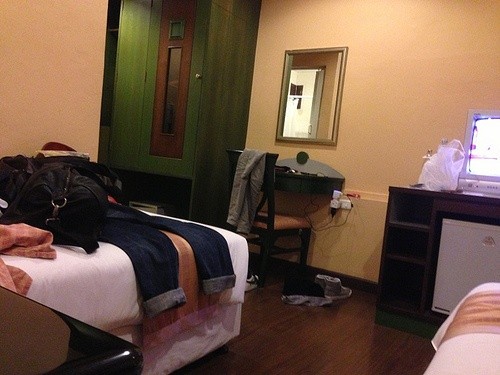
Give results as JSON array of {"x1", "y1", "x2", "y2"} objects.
[{"x1": 0, "y1": 164, "x2": 107, "y2": 255}]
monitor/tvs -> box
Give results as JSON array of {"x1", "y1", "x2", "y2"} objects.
[{"x1": 457, "y1": 109, "x2": 500, "y2": 198}]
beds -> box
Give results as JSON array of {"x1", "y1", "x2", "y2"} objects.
[
  {"x1": 0, "y1": 210, "x2": 248, "y2": 375},
  {"x1": 423, "y1": 283, "x2": 500, "y2": 375}
]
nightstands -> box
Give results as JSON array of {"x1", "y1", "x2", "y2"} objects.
[{"x1": 0, "y1": 285, "x2": 144, "y2": 375}]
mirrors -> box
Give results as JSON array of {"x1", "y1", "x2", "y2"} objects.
[{"x1": 275, "y1": 46, "x2": 349, "y2": 146}]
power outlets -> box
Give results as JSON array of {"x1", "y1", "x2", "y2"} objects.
[{"x1": 330, "y1": 199, "x2": 351, "y2": 210}]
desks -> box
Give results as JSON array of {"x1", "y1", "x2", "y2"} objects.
[{"x1": 374, "y1": 186, "x2": 500, "y2": 339}]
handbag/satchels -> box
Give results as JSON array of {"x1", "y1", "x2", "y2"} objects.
[{"x1": 418, "y1": 139, "x2": 465, "y2": 193}]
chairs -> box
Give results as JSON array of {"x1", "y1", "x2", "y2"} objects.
[{"x1": 226, "y1": 149, "x2": 310, "y2": 287}]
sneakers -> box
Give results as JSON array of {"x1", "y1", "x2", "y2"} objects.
[
  {"x1": 245, "y1": 275, "x2": 259, "y2": 292},
  {"x1": 315, "y1": 274, "x2": 352, "y2": 301}
]
